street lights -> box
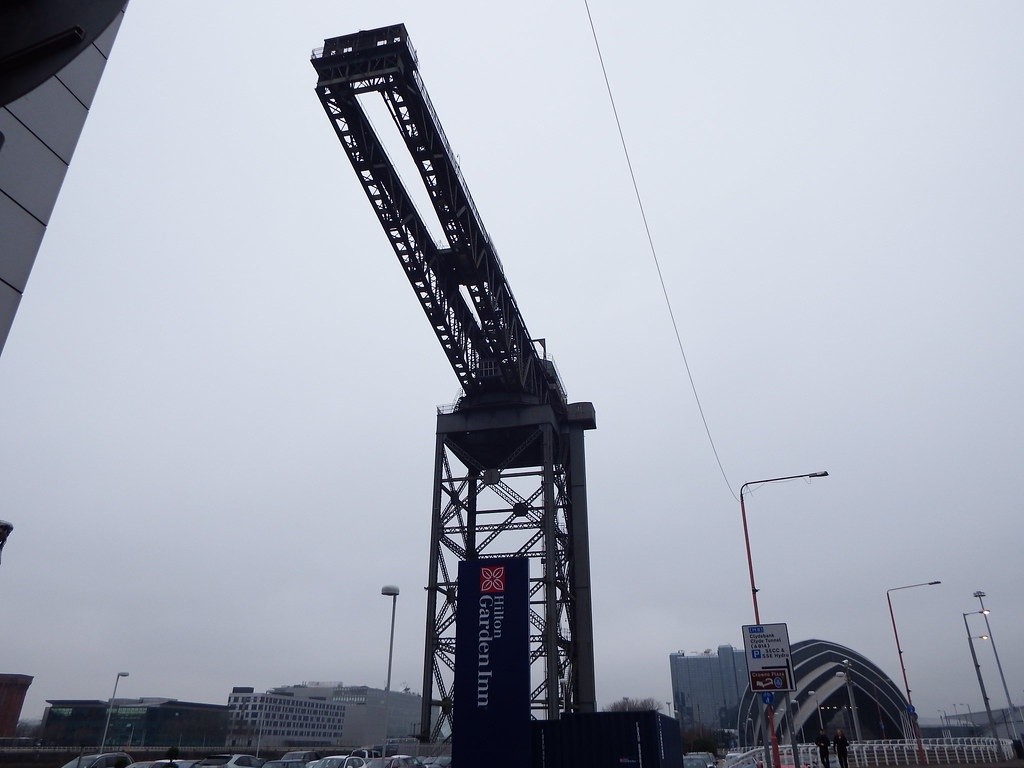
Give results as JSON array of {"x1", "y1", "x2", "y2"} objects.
[
  {"x1": 736, "y1": 470, "x2": 830, "y2": 623},
  {"x1": 961, "y1": 610, "x2": 1011, "y2": 764},
  {"x1": 380, "y1": 583, "x2": 399, "y2": 768},
  {"x1": 99, "y1": 671, "x2": 130, "y2": 750},
  {"x1": 972, "y1": 589, "x2": 1014, "y2": 709},
  {"x1": 884, "y1": 579, "x2": 944, "y2": 766}
]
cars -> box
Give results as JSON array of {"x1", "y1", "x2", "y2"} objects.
[
  {"x1": 60, "y1": 752, "x2": 134, "y2": 768},
  {"x1": 121, "y1": 751, "x2": 453, "y2": 768}
]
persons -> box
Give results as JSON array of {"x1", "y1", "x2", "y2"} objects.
[
  {"x1": 815, "y1": 728, "x2": 830, "y2": 768},
  {"x1": 833, "y1": 728, "x2": 849, "y2": 768}
]
